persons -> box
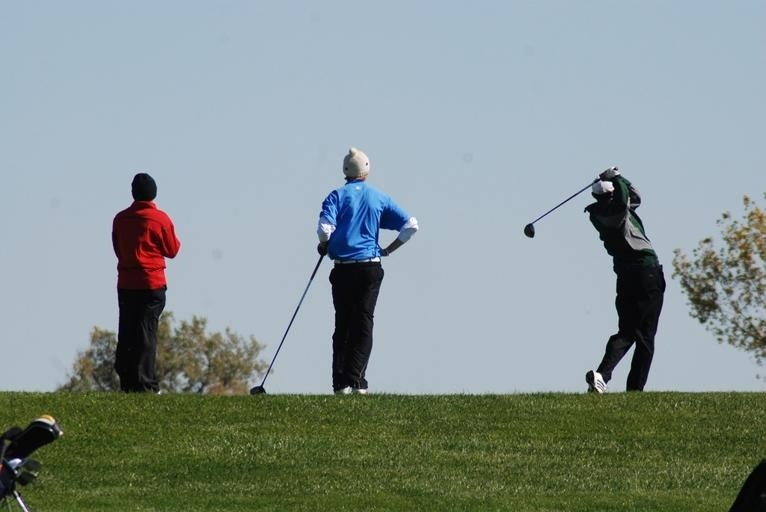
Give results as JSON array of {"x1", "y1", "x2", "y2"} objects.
[
  {"x1": 110, "y1": 172, "x2": 181, "y2": 395},
  {"x1": 582, "y1": 165, "x2": 667, "y2": 394},
  {"x1": 317, "y1": 147, "x2": 419, "y2": 395}
]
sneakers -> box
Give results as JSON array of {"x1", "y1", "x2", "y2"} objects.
[
  {"x1": 335, "y1": 386, "x2": 367, "y2": 395},
  {"x1": 586, "y1": 370, "x2": 608, "y2": 394}
]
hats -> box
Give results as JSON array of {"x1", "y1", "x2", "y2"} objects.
[
  {"x1": 592, "y1": 180, "x2": 615, "y2": 195},
  {"x1": 343, "y1": 147, "x2": 370, "y2": 178},
  {"x1": 131, "y1": 172, "x2": 157, "y2": 201}
]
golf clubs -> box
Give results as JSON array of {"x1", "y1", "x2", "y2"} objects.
[
  {"x1": 250, "y1": 256, "x2": 325, "y2": 395},
  {"x1": 523, "y1": 167, "x2": 619, "y2": 239},
  {"x1": 0, "y1": 415, "x2": 65, "y2": 512}
]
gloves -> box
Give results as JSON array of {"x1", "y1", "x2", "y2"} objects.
[
  {"x1": 600, "y1": 167, "x2": 621, "y2": 181},
  {"x1": 318, "y1": 241, "x2": 328, "y2": 257},
  {"x1": 379, "y1": 249, "x2": 390, "y2": 257}
]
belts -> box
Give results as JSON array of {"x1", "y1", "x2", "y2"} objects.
[{"x1": 334, "y1": 256, "x2": 381, "y2": 265}]
